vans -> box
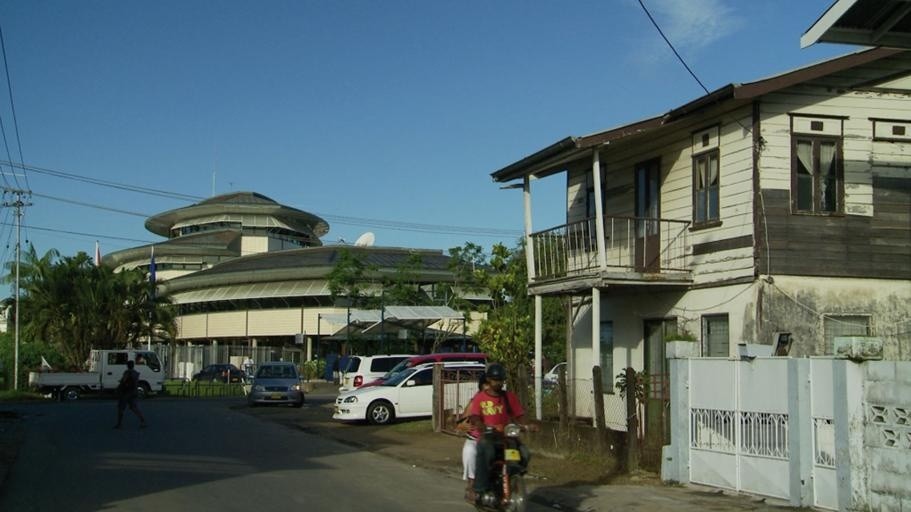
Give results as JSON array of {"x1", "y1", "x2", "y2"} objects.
[
  {"x1": 331, "y1": 355, "x2": 354, "y2": 386},
  {"x1": 337, "y1": 352, "x2": 423, "y2": 394},
  {"x1": 358, "y1": 351, "x2": 495, "y2": 390}
]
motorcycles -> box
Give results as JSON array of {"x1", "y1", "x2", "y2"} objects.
[{"x1": 461, "y1": 424, "x2": 534, "y2": 511}]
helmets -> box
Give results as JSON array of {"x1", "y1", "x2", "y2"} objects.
[
  {"x1": 488, "y1": 365, "x2": 507, "y2": 380},
  {"x1": 479, "y1": 374, "x2": 489, "y2": 388}
]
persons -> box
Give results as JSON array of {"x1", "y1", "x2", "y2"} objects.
[
  {"x1": 135, "y1": 353, "x2": 148, "y2": 364},
  {"x1": 462, "y1": 372, "x2": 493, "y2": 501},
  {"x1": 464, "y1": 363, "x2": 541, "y2": 501},
  {"x1": 243, "y1": 356, "x2": 255, "y2": 375},
  {"x1": 113, "y1": 360, "x2": 151, "y2": 430}
]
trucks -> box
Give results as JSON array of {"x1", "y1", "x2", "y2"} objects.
[{"x1": 27, "y1": 348, "x2": 166, "y2": 401}]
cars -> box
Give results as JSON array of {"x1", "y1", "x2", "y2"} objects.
[
  {"x1": 330, "y1": 358, "x2": 507, "y2": 426},
  {"x1": 543, "y1": 361, "x2": 568, "y2": 384},
  {"x1": 250, "y1": 360, "x2": 305, "y2": 408},
  {"x1": 190, "y1": 364, "x2": 246, "y2": 383}
]
